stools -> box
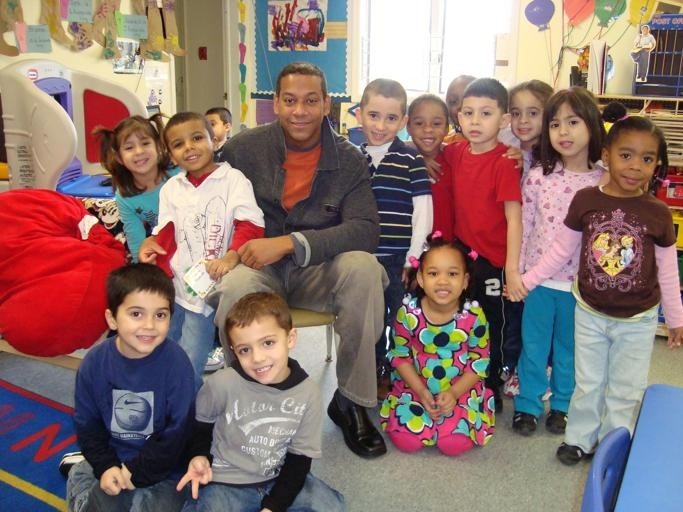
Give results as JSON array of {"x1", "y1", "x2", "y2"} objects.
[{"x1": 288, "y1": 307, "x2": 338, "y2": 362}]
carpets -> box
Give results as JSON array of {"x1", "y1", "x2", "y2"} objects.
[
  {"x1": 0, "y1": 376, "x2": 87, "y2": 512},
  {"x1": 1, "y1": 330, "x2": 110, "y2": 371}
]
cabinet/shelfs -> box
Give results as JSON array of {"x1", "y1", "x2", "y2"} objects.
[{"x1": 594, "y1": 93, "x2": 682, "y2": 338}]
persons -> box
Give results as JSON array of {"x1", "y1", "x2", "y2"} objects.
[
  {"x1": 630, "y1": 25, "x2": 657, "y2": 82},
  {"x1": 90, "y1": 60, "x2": 682, "y2": 465},
  {"x1": 59, "y1": 261, "x2": 197, "y2": 510},
  {"x1": 174, "y1": 289, "x2": 349, "y2": 511}
]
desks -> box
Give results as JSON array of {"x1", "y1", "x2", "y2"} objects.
[{"x1": 612, "y1": 384, "x2": 683, "y2": 512}]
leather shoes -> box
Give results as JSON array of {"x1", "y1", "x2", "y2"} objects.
[{"x1": 327, "y1": 388, "x2": 387, "y2": 459}]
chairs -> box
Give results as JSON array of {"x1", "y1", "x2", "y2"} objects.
[{"x1": 580, "y1": 425, "x2": 632, "y2": 512}]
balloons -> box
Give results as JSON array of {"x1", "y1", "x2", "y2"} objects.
[{"x1": 524, "y1": 1, "x2": 658, "y2": 30}]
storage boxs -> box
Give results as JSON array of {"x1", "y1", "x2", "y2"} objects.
[{"x1": 651, "y1": 165, "x2": 682, "y2": 324}]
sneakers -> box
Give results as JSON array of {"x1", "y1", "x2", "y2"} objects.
[
  {"x1": 556, "y1": 442, "x2": 582, "y2": 464},
  {"x1": 502, "y1": 372, "x2": 519, "y2": 397},
  {"x1": 513, "y1": 412, "x2": 536, "y2": 435},
  {"x1": 546, "y1": 411, "x2": 568, "y2": 434},
  {"x1": 59, "y1": 452, "x2": 84, "y2": 478}
]
